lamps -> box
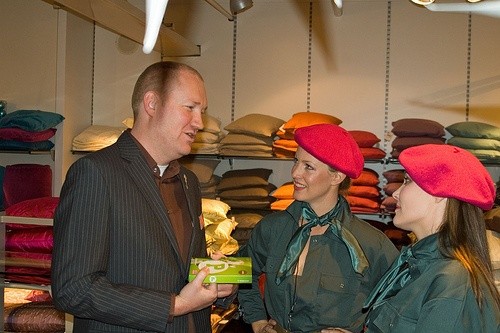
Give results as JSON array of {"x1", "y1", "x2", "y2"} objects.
[{"x1": 228, "y1": 0, "x2": 254, "y2": 16}]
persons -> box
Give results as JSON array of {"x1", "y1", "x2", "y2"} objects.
[
  {"x1": 237, "y1": 124, "x2": 399, "y2": 333},
  {"x1": 52, "y1": 61, "x2": 236, "y2": 333},
  {"x1": 363, "y1": 144, "x2": 500, "y2": 333}
]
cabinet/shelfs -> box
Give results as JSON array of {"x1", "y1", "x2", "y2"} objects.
[
  {"x1": 0, "y1": 149, "x2": 55, "y2": 296},
  {"x1": 0, "y1": 216, "x2": 56, "y2": 291}
]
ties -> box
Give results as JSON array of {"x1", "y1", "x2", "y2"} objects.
[
  {"x1": 362, "y1": 247, "x2": 415, "y2": 327},
  {"x1": 274, "y1": 198, "x2": 370, "y2": 287}
]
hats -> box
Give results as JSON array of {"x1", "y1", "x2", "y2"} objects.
[
  {"x1": 293, "y1": 123, "x2": 365, "y2": 180},
  {"x1": 399, "y1": 143, "x2": 496, "y2": 212}
]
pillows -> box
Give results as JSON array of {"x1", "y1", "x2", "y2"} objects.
[
  {"x1": 0, "y1": 110, "x2": 65, "y2": 154},
  {"x1": 0, "y1": 163, "x2": 67, "y2": 333},
  {"x1": 3, "y1": 303, "x2": 66, "y2": 333},
  {"x1": 72, "y1": 119, "x2": 500, "y2": 283}
]
